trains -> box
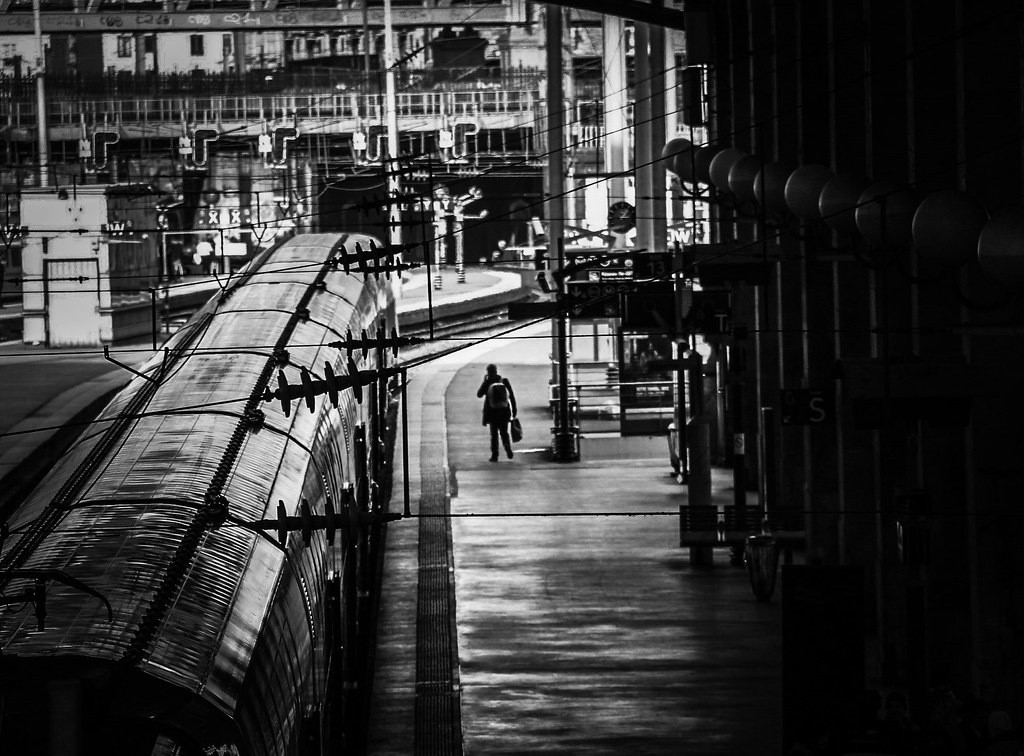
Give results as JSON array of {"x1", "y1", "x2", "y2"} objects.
[{"x1": 1, "y1": 225, "x2": 408, "y2": 755}]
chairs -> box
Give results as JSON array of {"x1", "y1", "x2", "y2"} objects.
[{"x1": 679, "y1": 502, "x2": 806, "y2": 565}]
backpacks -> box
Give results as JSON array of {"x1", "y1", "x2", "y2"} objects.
[{"x1": 488, "y1": 377, "x2": 509, "y2": 410}]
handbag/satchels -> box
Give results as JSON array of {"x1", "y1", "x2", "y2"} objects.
[{"x1": 511, "y1": 418, "x2": 522, "y2": 443}]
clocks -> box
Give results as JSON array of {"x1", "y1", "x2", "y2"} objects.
[{"x1": 609, "y1": 201, "x2": 636, "y2": 234}]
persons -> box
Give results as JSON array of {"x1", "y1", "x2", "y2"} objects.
[
  {"x1": 171, "y1": 233, "x2": 225, "y2": 280},
  {"x1": 477, "y1": 364, "x2": 517, "y2": 462}
]
